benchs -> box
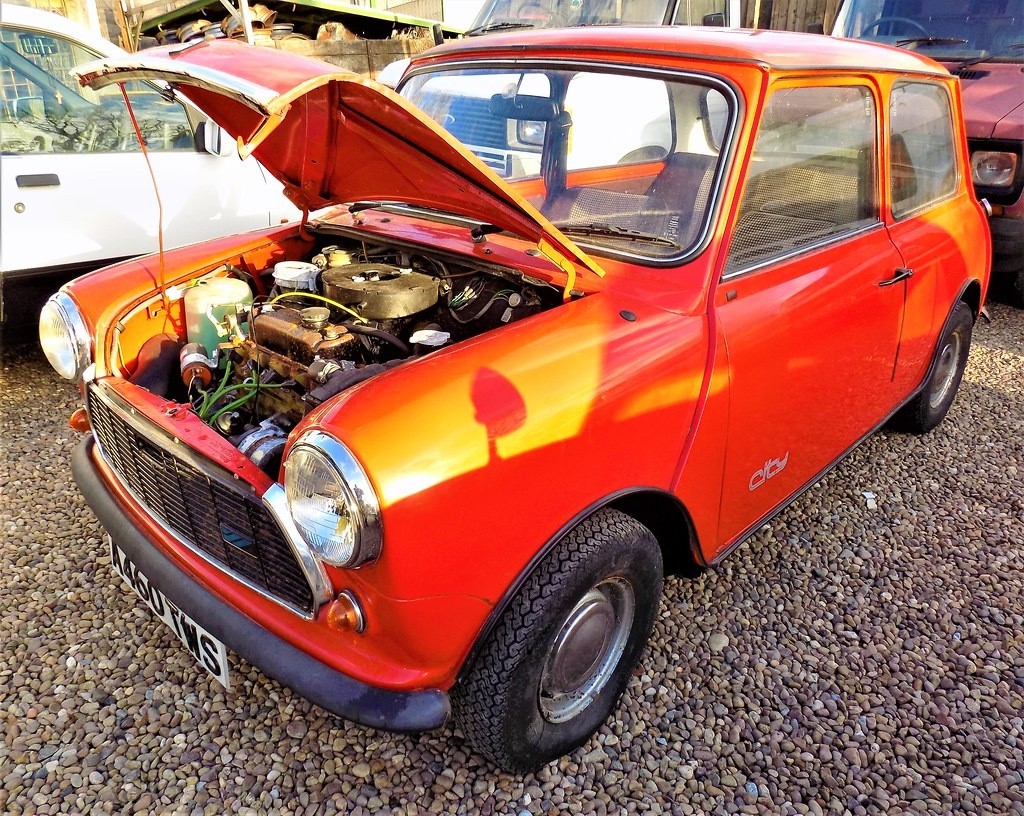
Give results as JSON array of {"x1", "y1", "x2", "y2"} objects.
[{"x1": 649, "y1": 148, "x2": 868, "y2": 237}]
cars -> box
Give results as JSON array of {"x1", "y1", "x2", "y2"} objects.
[
  {"x1": 0, "y1": 3, "x2": 353, "y2": 345},
  {"x1": 374, "y1": 1, "x2": 743, "y2": 180},
  {"x1": 38, "y1": 24, "x2": 994, "y2": 775},
  {"x1": 760, "y1": 0, "x2": 1024, "y2": 276}
]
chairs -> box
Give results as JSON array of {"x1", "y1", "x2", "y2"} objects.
[
  {"x1": 903, "y1": 0, "x2": 984, "y2": 50},
  {"x1": 543, "y1": 182, "x2": 664, "y2": 238},
  {"x1": 721, "y1": 209, "x2": 842, "y2": 274}
]
